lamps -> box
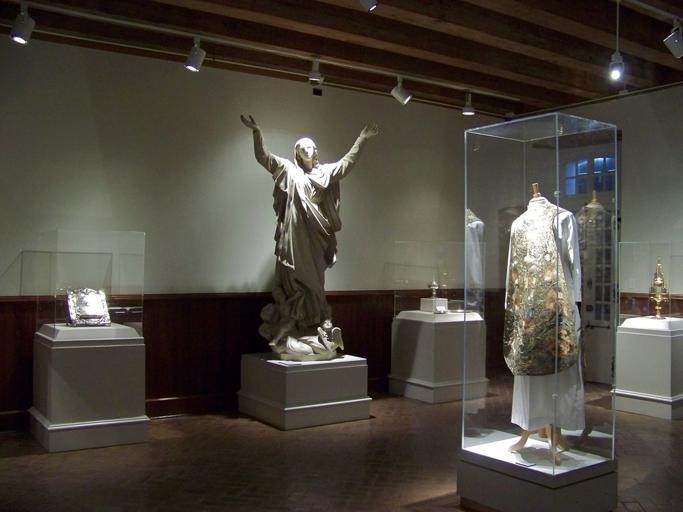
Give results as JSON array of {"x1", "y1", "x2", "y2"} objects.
[
  {"x1": 390, "y1": 75, "x2": 414, "y2": 106},
  {"x1": 605, "y1": 53, "x2": 631, "y2": 88},
  {"x1": 360, "y1": 1, "x2": 378, "y2": 13},
  {"x1": 11, "y1": 1, "x2": 36, "y2": 46},
  {"x1": 461, "y1": 90, "x2": 475, "y2": 116},
  {"x1": 305, "y1": 59, "x2": 324, "y2": 87},
  {"x1": 184, "y1": 37, "x2": 207, "y2": 73}
]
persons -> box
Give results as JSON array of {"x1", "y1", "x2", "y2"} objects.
[
  {"x1": 465, "y1": 207, "x2": 488, "y2": 415},
  {"x1": 266, "y1": 285, "x2": 303, "y2": 347},
  {"x1": 500, "y1": 182, "x2": 586, "y2": 451},
  {"x1": 239, "y1": 112, "x2": 380, "y2": 337}
]
